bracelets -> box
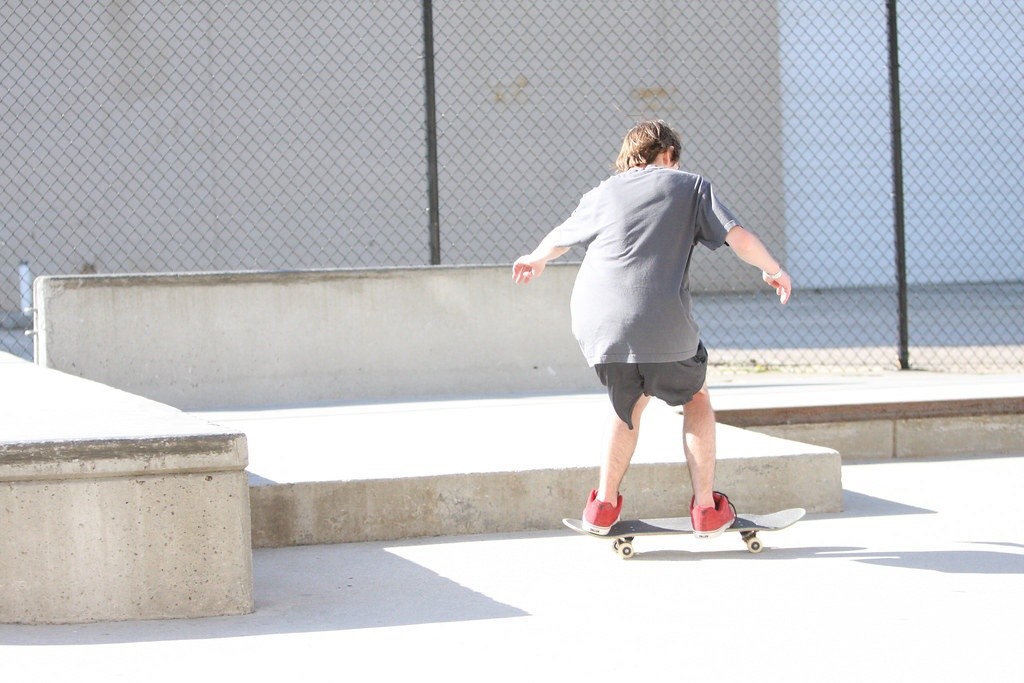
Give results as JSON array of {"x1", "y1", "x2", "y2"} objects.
[{"x1": 763, "y1": 268, "x2": 783, "y2": 279}]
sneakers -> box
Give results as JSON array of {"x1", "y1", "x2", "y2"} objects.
[
  {"x1": 582, "y1": 488, "x2": 623, "y2": 535},
  {"x1": 689, "y1": 491, "x2": 738, "y2": 537}
]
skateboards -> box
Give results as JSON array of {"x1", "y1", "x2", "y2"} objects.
[{"x1": 563, "y1": 508, "x2": 808, "y2": 560}]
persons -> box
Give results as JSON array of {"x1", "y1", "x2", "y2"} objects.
[{"x1": 510, "y1": 120, "x2": 792, "y2": 537}]
